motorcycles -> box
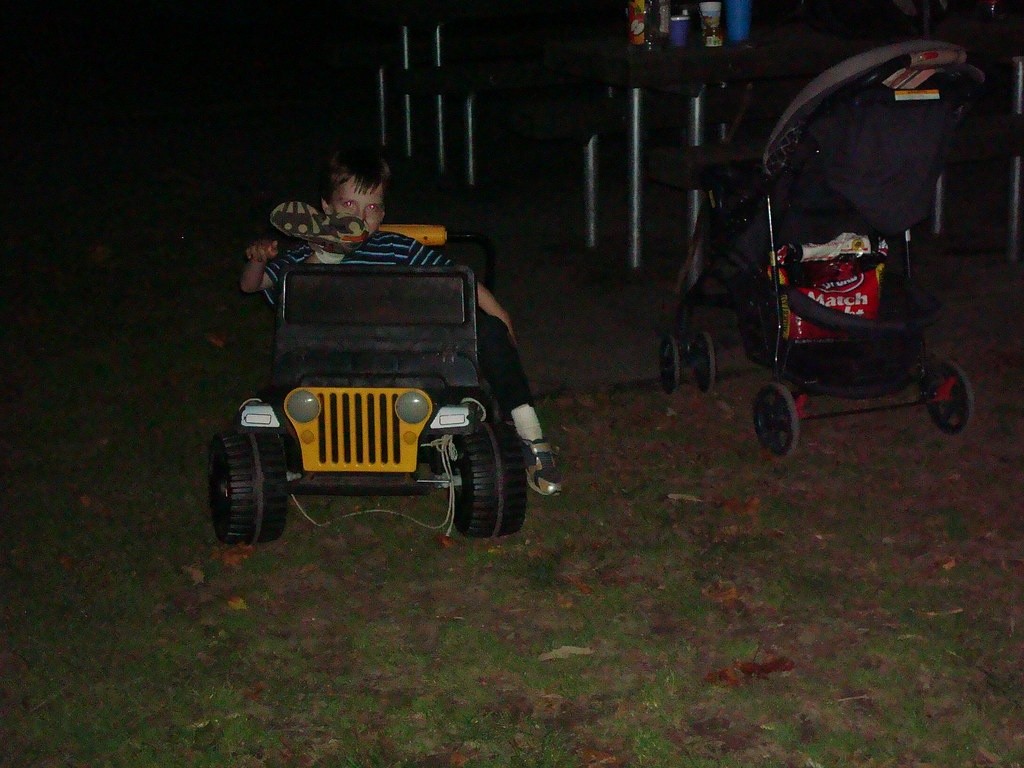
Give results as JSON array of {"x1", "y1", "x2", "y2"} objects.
[{"x1": 209, "y1": 224, "x2": 528, "y2": 543}]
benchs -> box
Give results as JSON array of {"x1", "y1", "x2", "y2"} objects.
[{"x1": 355, "y1": 55, "x2": 1024, "y2": 299}]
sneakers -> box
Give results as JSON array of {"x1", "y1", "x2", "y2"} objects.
[
  {"x1": 270, "y1": 202, "x2": 369, "y2": 257},
  {"x1": 517, "y1": 434, "x2": 562, "y2": 496}
]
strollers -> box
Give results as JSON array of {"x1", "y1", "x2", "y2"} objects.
[{"x1": 658, "y1": 39, "x2": 974, "y2": 455}]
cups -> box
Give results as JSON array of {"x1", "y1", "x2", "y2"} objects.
[
  {"x1": 670, "y1": 15, "x2": 690, "y2": 47},
  {"x1": 725, "y1": 0, "x2": 751, "y2": 41},
  {"x1": 699, "y1": 2, "x2": 721, "y2": 28}
]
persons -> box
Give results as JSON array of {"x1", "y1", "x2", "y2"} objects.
[{"x1": 237, "y1": 152, "x2": 563, "y2": 498}]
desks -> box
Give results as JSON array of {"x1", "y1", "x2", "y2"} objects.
[
  {"x1": 357, "y1": 28, "x2": 619, "y2": 179},
  {"x1": 563, "y1": 17, "x2": 1024, "y2": 300}
]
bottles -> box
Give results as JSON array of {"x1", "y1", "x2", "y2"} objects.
[
  {"x1": 698, "y1": 26, "x2": 723, "y2": 46},
  {"x1": 627, "y1": 0, "x2": 671, "y2": 46}
]
